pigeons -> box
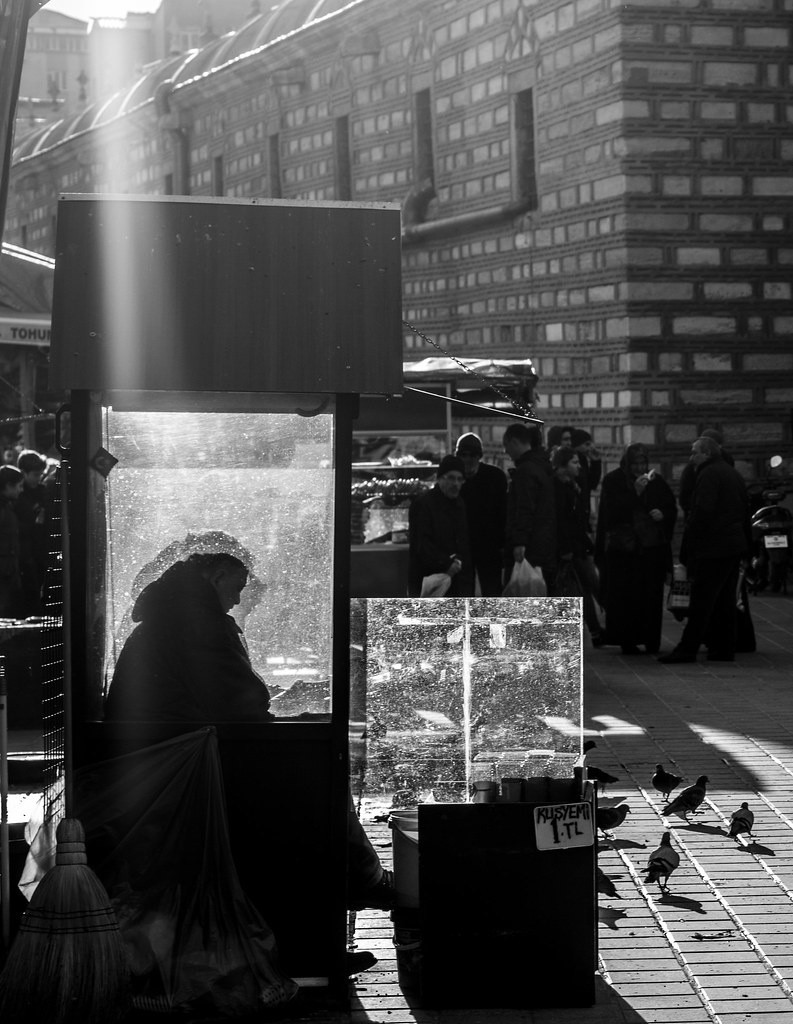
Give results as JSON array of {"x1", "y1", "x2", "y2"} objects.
[
  {"x1": 726, "y1": 802, "x2": 756, "y2": 841},
  {"x1": 640, "y1": 832, "x2": 679, "y2": 889},
  {"x1": 660, "y1": 775, "x2": 710, "y2": 825},
  {"x1": 597, "y1": 804, "x2": 631, "y2": 837},
  {"x1": 598, "y1": 867, "x2": 622, "y2": 899},
  {"x1": 586, "y1": 766, "x2": 620, "y2": 793},
  {"x1": 651, "y1": 764, "x2": 683, "y2": 802},
  {"x1": 584, "y1": 740, "x2": 597, "y2": 753}
]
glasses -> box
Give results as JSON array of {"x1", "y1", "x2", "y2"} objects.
[{"x1": 442, "y1": 472, "x2": 467, "y2": 487}]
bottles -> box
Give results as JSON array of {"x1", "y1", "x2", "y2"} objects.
[{"x1": 473, "y1": 777, "x2": 578, "y2": 803}]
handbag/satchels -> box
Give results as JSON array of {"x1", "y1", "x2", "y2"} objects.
[
  {"x1": 502, "y1": 559, "x2": 546, "y2": 599},
  {"x1": 666, "y1": 562, "x2": 689, "y2": 622}
]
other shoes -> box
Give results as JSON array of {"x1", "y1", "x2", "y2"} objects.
[
  {"x1": 657, "y1": 648, "x2": 698, "y2": 665},
  {"x1": 702, "y1": 647, "x2": 734, "y2": 662},
  {"x1": 731, "y1": 643, "x2": 758, "y2": 654},
  {"x1": 592, "y1": 628, "x2": 607, "y2": 648},
  {"x1": 346, "y1": 869, "x2": 396, "y2": 912},
  {"x1": 621, "y1": 643, "x2": 644, "y2": 656},
  {"x1": 281, "y1": 943, "x2": 376, "y2": 980},
  {"x1": 647, "y1": 643, "x2": 661, "y2": 657}
]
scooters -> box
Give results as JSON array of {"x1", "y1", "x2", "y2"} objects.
[{"x1": 743, "y1": 487, "x2": 793, "y2": 590}]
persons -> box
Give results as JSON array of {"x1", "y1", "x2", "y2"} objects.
[
  {"x1": 502, "y1": 424, "x2": 567, "y2": 600},
  {"x1": 546, "y1": 427, "x2": 757, "y2": 665},
  {"x1": 408, "y1": 432, "x2": 509, "y2": 597},
  {"x1": 102, "y1": 529, "x2": 396, "y2": 975},
  {"x1": 0, "y1": 443, "x2": 64, "y2": 730}
]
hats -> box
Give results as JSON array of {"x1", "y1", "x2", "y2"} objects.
[
  {"x1": 456, "y1": 435, "x2": 484, "y2": 459},
  {"x1": 436, "y1": 454, "x2": 464, "y2": 478}
]
